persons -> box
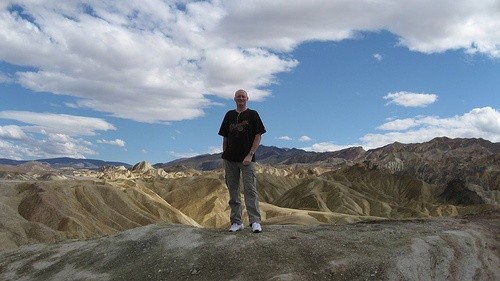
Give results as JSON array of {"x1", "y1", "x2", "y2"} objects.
[{"x1": 218, "y1": 88, "x2": 266, "y2": 234}]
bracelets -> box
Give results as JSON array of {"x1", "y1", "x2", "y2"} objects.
[{"x1": 249, "y1": 151, "x2": 254, "y2": 156}]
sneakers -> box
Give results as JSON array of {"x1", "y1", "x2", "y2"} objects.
[
  {"x1": 229, "y1": 223, "x2": 245, "y2": 232},
  {"x1": 251, "y1": 222, "x2": 263, "y2": 232}
]
